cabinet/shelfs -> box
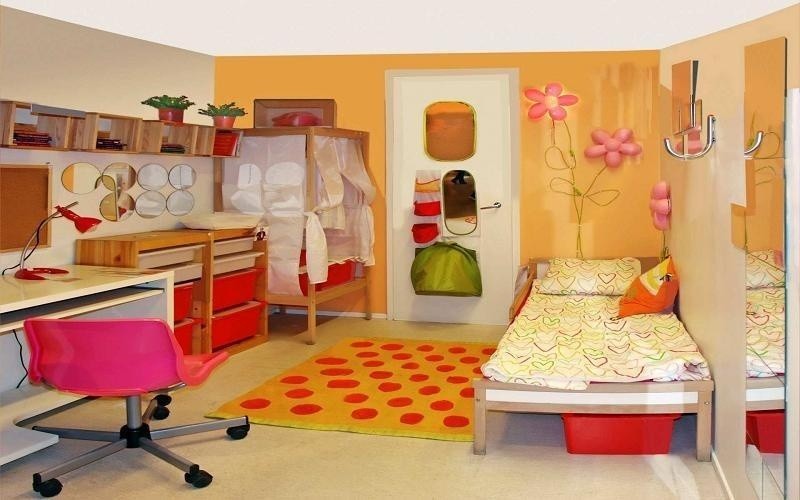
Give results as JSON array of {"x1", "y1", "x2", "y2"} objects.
[
  {"x1": 72, "y1": 226, "x2": 271, "y2": 354},
  {"x1": 0, "y1": 100, "x2": 244, "y2": 159},
  {"x1": 211, "y1": 127, "x2": 372, "y2": 346}
]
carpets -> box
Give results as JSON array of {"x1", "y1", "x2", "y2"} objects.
[{"x1": 200, "y1": 334, "x2": 500, "y2": 442}]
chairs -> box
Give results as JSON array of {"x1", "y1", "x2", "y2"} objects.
[{"x1": 24, "y1": 313, "x2": 254, "y2": 498}]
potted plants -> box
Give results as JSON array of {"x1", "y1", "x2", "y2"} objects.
[
  {"x1": 140, "y1": 94, "x2": 197, "y2": 123},
  {"x1": 196, "y1": 102, "x2": 250, "y2": 128}
]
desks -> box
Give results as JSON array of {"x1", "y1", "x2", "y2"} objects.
[{"x1": 1, "y1": 264, "x2": 176, "y2": 469}]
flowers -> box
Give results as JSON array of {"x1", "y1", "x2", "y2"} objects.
[{"x1": 521, "y1": 78, "x2": 644, "y2": 259}]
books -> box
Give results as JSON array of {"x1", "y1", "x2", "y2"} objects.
[
  {"x1": 213, "y1": 131, "x2": 243, "y2": 157},
  {"x1": 160, "y1": 142, "x2": 185, "y2": 153},
  {"x1": 13, "y1": 128, "x2": 52, "y2": 147},
  {"x1": 96, "y1": 139, "x2": 128, "y2": 150}
]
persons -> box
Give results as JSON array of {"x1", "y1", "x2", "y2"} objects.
[{"x1": 451, "y1": 171, "x2": 467, "y2": 184}]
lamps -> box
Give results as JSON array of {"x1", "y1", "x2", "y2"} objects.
[{"x1": 13, "y1": 201, "x2": 104, "y2": 282}]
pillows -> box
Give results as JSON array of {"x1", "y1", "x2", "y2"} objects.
[
  {"x1": 746, "y1": 250, "x2": 788, "y2": 291},
  {"x1": 609, "y1": 253, "x2": 681, "y2": 321},
  {"x1": 536, "y1": 257, "x2": 643, "y2": 297}
]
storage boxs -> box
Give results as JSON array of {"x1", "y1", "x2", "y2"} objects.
[
  {"x1": 556, "y1": 411, "x2": 683, "y2": 455},
  {"x1": 137, "y1": 235, "x2": 269, "y2": 355},
  {"x1": 744, "y1": 408, "x2": 785, "y2": 457}
]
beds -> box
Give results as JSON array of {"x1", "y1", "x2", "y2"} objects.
[
  {"x1": 469, "y1": 257, "x2": 716, "y2": 462},
  {"x1": 743, "y1": 246, "x2": 786, "y2": 413}
]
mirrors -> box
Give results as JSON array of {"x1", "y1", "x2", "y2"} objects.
[
  {"x1": 741, "y1": 35, "x2": 790, "y2": 500},
  {"x1": 668, "y1": 58, "x2": 696, "y2": 139}
]
